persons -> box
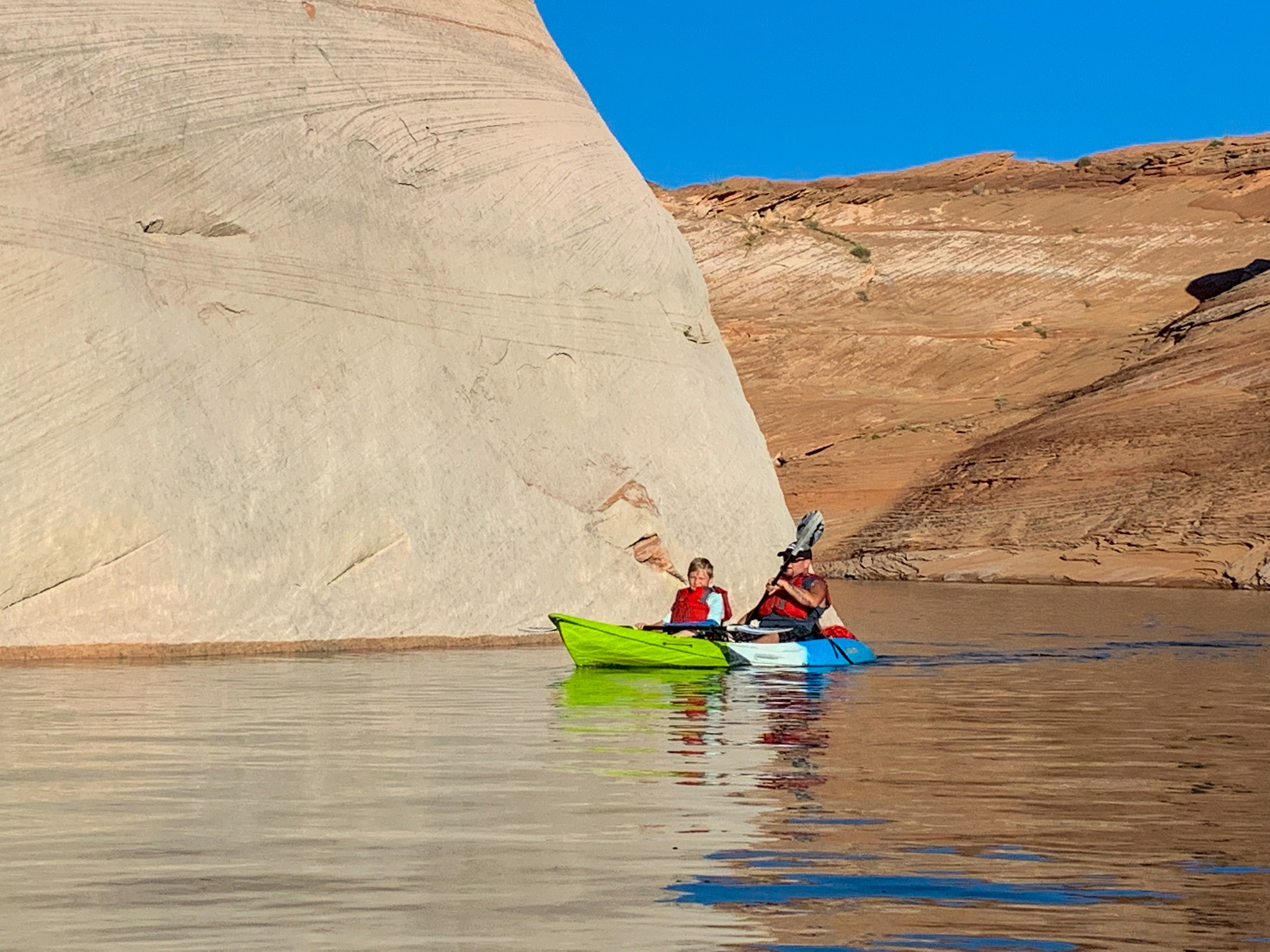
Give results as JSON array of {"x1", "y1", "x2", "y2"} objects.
[
  {"x1": 729, "y1": 541, "x2": 832, "y2": 644},
  {"x1": 635, "y1": 557, "x2": 725, "y2": 638}
]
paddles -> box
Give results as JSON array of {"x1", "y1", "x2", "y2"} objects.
[
  {"x1": 519, "y1": 624, "x2": 794, "y2": 635},
  {"x1": 745, "y1": 509, "x2": 825, "y2": 623}
]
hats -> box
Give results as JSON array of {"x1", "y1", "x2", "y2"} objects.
[{"x1": 777, "y1": 542, "x2": 813, "y2": 559}]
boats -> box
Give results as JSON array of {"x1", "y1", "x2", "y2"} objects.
[{"x1": 547, "y1": 612, "x2": 876, "y2": 670}]
